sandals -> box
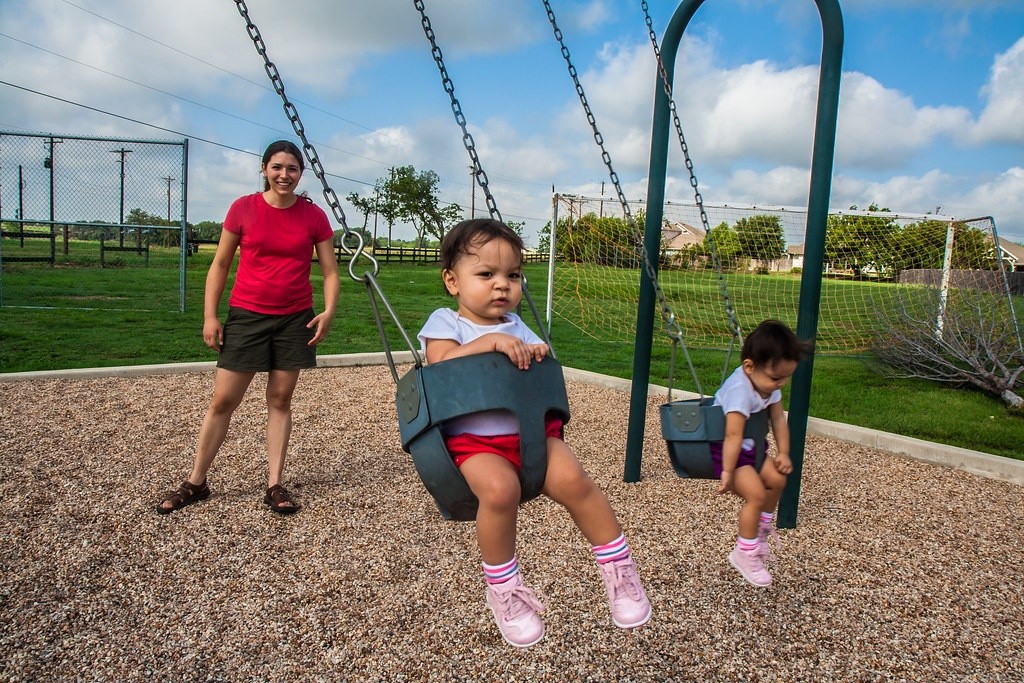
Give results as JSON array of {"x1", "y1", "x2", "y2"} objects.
[
  {"x1": 264, "y1": 484, "x2": 296, "y2": 514},
  {"x1": 156, "y1": 476, "x2": 210, "y2": 515}
]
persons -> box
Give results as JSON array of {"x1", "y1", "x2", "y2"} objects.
[
  {"x1": 156, "y1": 140, "x2": 340, "y2": 513},
  {"x1": 713, "y1": 318, "x2": 800, "y2": 588},
  {"x1": 418, "y1": 217, "x2": 654, "y2": 648}
]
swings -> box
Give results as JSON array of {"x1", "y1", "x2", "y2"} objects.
[
  {"x1": 231, "y1": 0, "x2": 572, "y2": 522},
  {"x1": 540, "y1": 0, "x2": 772, "y2": 482}
]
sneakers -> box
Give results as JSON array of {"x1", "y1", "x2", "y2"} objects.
[
  {"x1": 728, "y1": 546, "x2": 773, "y2": 587},
  {"x1": 485, "y1": 574, "x2": 546, "y2": 649},
  {"x1": 757, "y1": 522, "x2": 777, "y2": 556},
  {"x1": 598, "y1": 553, "x2": 652, "y2": 629}
]
